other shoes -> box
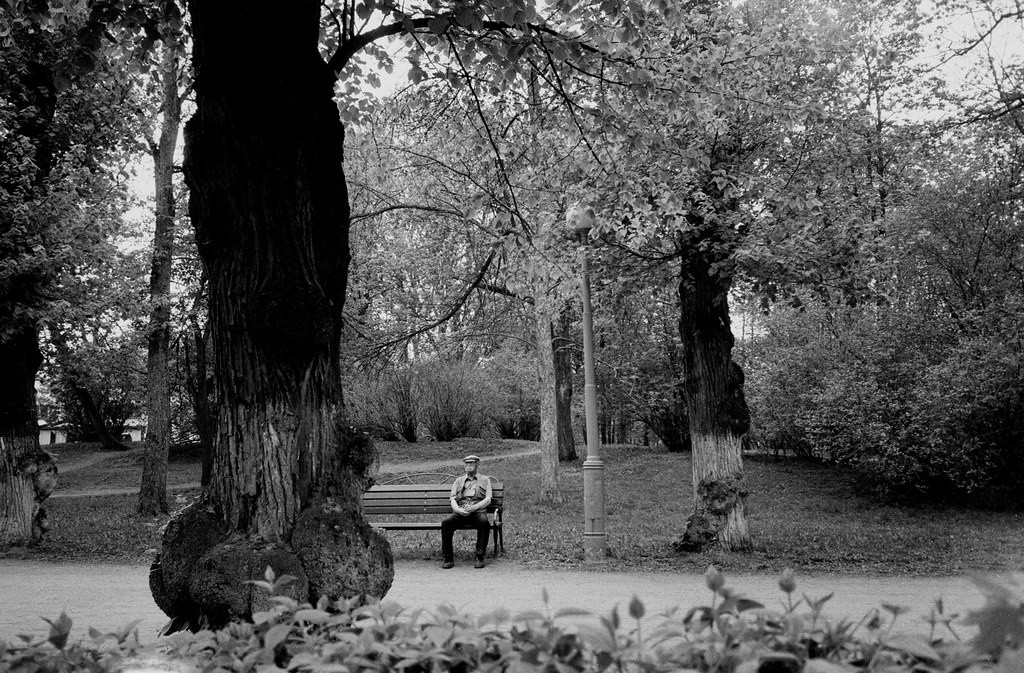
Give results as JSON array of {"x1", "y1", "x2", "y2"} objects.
[
  {"x1": 442, "y1": 556, "x2": 454, "y2": 568},
  {"x1": 475, "y1": 555, "x2": 484, "y2": 568}
]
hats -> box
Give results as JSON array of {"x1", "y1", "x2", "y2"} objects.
[{"x1": 463, "y1": 455, "x2": 479, "y2": 463}]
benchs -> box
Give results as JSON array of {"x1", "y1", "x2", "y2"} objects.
[{"x1": 358, "y1": 482, "x2": 508, "y2": 558}]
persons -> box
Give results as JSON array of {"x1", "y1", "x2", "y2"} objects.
[{"x1": 441, "y1": 455, "x2": 492, "y2": 570}]
